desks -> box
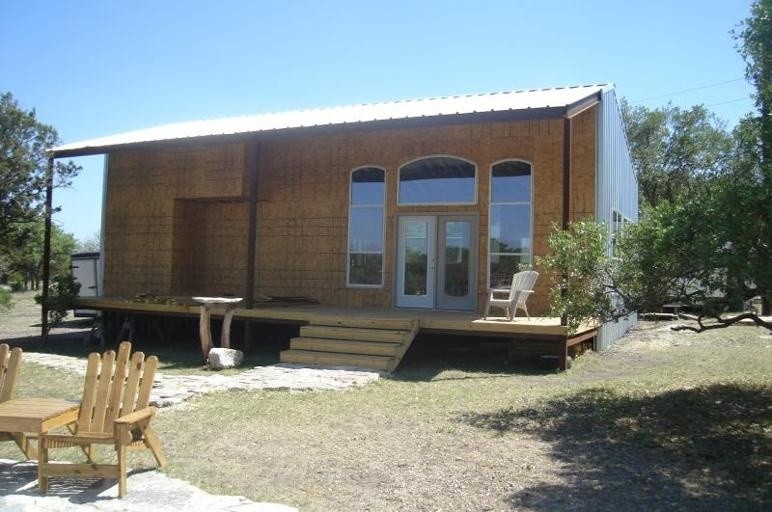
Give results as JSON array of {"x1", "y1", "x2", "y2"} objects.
[{"x1": 1, "y1": 394, "x2": 102, "y2": 464}]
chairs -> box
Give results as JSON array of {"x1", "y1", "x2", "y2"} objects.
[
  {"x1": 482, "y1": 269, "x2": 541, "y2": 322},
  {"x1": 37, "y1": 340, "x2": 167, "y2": 498}
]
